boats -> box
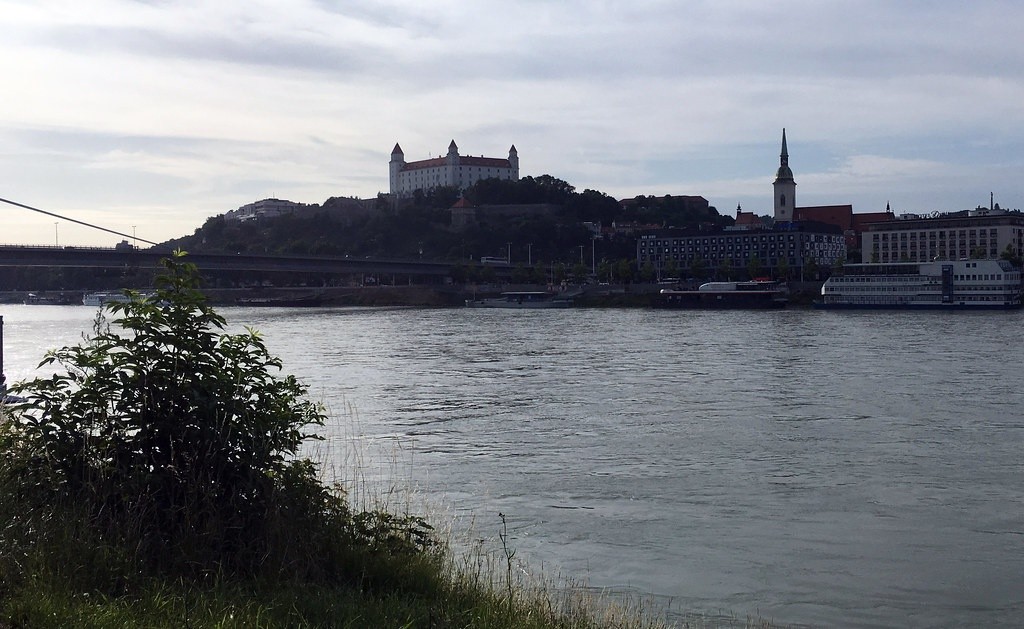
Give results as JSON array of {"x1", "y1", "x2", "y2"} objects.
[
  {"x1": 820, "y1": 258, "x2": 1022, "y2": 309},
  {"x1": 659, "y1": 276, "x2": 790, "y2": 309},
  {"x1": 465, "y1": 291, "x2": 576, "y2": 309}
]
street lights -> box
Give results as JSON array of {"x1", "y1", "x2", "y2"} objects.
[
  {"x1": 506, "y1": 242, "x2": 512, "y2": 263},
  {"x1": 55, "y1": 221, "x2": 59, "y2": 247},
  {"x1": 527, "y1": 243, "x2": 533, "y2": 266},
  {"x1": 579, "y1": 246, "x2": 585, "y2": 264},
  {"x1": 131, "y1": 226, "x2": 137, "y2": 247},
  {"x1": 590, "y1": 238, "x2": 597, "y2": 276}
]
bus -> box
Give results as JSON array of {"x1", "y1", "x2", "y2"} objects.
[
  {"x1": 481, "y1": 256, "x2": 508, "y2": 263},
  {"x1": 657, "y1": 277, "x2": 681, "y2": 285}
]
